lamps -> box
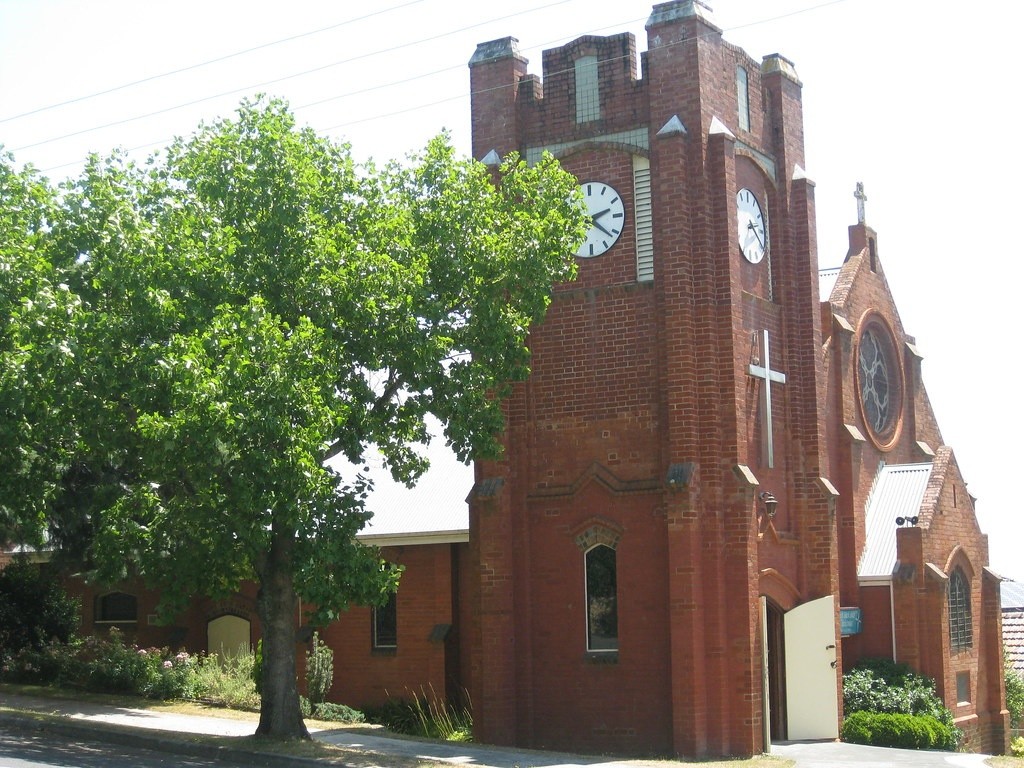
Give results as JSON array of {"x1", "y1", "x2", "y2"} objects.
[{"x1": 760, "y1": 492, "x2": 779, "y2": 518}]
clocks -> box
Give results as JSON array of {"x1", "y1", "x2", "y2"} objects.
[
  {"x1": 562, "y1": 182, "x2": 625, "y2": 258},
  {"x1": 736, "y1": 186, "x2": 766, "y2": 265}
]
